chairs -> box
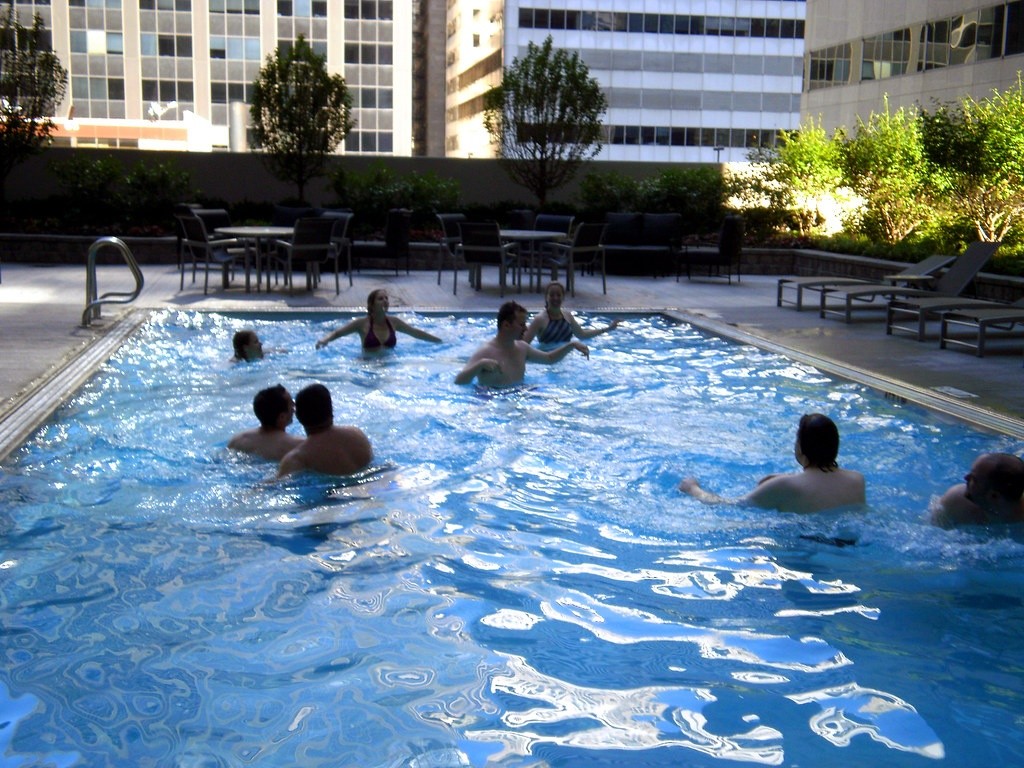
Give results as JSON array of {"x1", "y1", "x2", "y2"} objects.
[
  {"x1": 169, "y1": 199, "x2": 743, "y2": 299},
  {"x1": 777, "y1": 240, "x2": 1023, "y2": 358}
]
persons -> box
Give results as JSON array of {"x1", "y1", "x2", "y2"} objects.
[
  {"x1": 315, "y1": 288, "x2": 443, "y2": 354},
  {"x1": 929, "y1": 452, "x2": 1024, "y2": 530},
  {"x1": 522, "y1": 282, "x2": 620, "y2": 345},
  {"x1": 278, "y1": 383, "x2": 374, "y2": 477},
  {"x1": 679, "y1": 413, "x2": 865, "y2": 515},
  {"x1": 453, "y1": 301, "x2": 590, "y2": 386},
  {"x1": 226, "y1": 385, "x2": 303, "y2": 462},
  {"x1": 232, "y1": 329, "x2": 291, "y2": 361}
]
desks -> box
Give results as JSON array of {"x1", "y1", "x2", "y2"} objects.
[
  {"x1": 214, "y1": 226, "x2": 295, "y2": 292},
  {"x1": 499, "y1": 229, "x2": 567, "y2": 293}
]
